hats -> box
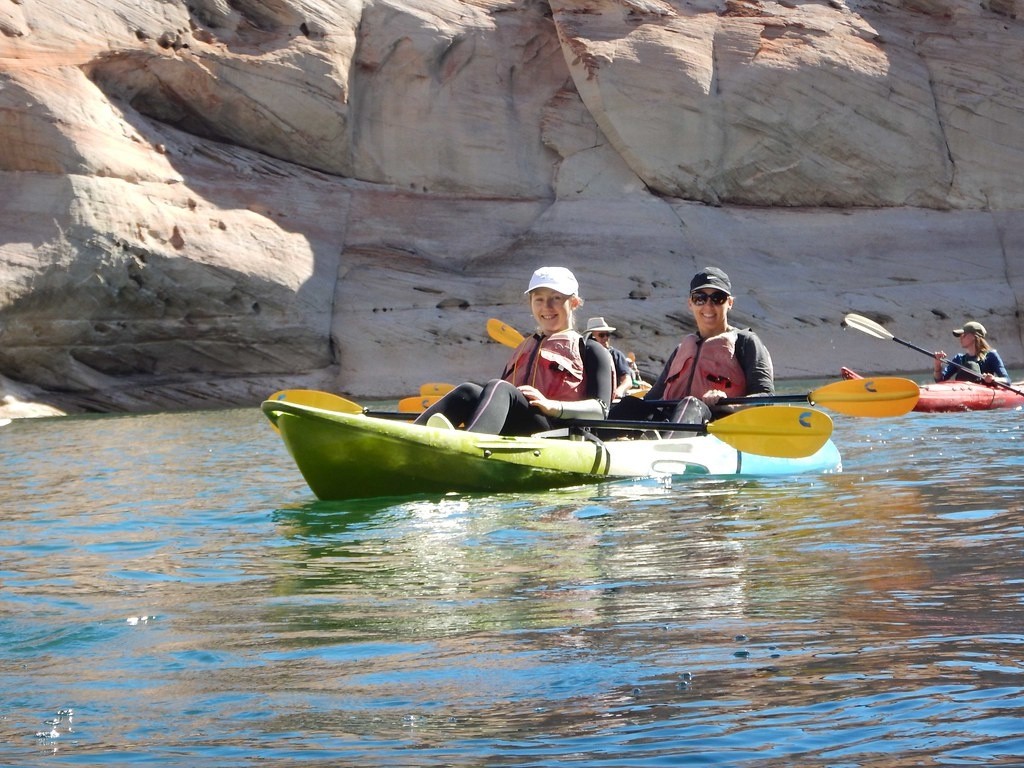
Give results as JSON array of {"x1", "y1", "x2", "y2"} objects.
[
  {"x1": 583, "y1": 317, "x2": 616, "y2": 332},
  {"x1": 690, "y1": 267, "x2": 732, "y2": 296},
  {"x1": 524, "y1": 267, "x2": 579, "y2": 298},
  {"x1": 953, "y1": 321, "x2": 986, "y2": 338}
]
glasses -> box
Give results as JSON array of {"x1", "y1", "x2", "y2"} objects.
[
  {"x1": 592, "y1": 332, "x2": 610, "y2": 338},
  {"x1": 691, "y1": 290, "x2": 728, "y2": 306}
]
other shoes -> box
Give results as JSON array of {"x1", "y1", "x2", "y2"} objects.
[
  {"x1": 638, "y1": 430, "x2": 662, "y2": 440},
  {"x1": 427, "y1": 412, "x2": 456, "y2": 432}
]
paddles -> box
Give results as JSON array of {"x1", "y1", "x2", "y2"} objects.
[
  {"x1": 843, "y1": 312, "x2": 1024, "y2": 397},
  {"x1": 396, "y1": 381, "x2": 465, "y2": 427},
  {"x1": 628, "y1": 351, "x2": 643, "y2": 384},
  {"x1": 610, "y1": 375, "x2": 920, "y2": 418},
  {"x1": 266, "y1": 388, "x2": 836, "y2": 461},
  {"x1": 485, "y1": 316, "x2": 526, "y2": 348}
]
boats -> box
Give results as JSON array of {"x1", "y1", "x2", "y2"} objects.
[
  {"x1": 259, "y1": 402, "x2": 843, "y2": 509},
  {"x1": 838, "y1": 364, "x2": 1024, "y2": 412}
]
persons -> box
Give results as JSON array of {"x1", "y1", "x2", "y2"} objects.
[
  {"x1": 414, "y1": 266, "x2": 616, "y2": 441},
  {"x1": 933, "y1": 321, "x2": 1012, "y2": 386},
  {"x1": 581, "y1": 318, "x2": 634, "y2": 407},
  {"x1": 600, "y1": 267, "x2": 774, "y2": 443}
]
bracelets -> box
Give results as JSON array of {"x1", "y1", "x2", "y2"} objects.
[{"x1": 935, "y1": 369, "x2": 941, "y2": 372}]
